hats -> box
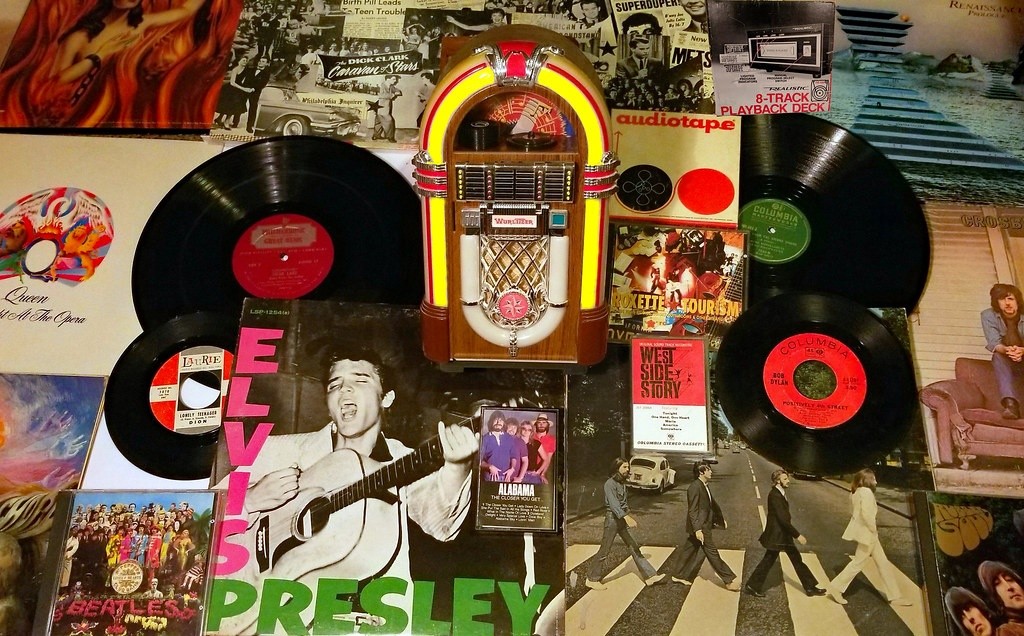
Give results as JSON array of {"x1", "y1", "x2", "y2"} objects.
[{"x1": 531, "y1": 414, "x2": 553, "y2": 427}]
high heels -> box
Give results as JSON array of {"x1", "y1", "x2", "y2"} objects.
[{"x1": 214, "y1": 119, "x2": 230, "y2": 130}]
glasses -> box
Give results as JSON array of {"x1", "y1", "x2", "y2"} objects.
[{"x1": 521, "y1": 427, "x2": 532, "y2": 432}]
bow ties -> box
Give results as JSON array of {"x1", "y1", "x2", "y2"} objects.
[{"x1": 256, "y1": 68, "x2": 261, "y2": 71}]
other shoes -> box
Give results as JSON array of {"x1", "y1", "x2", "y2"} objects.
[
  {"x1": 232, "y1": 123, "x2": 238, "y2": 127},
  {"x1": 726, "y1": 581, "x2": 741, "y2": 591},
  {"x1": 645, "y1": 573, "x2": 666, "y2": 585},
  {"x1": 247, "y1": 128, "x2": 253, "y2": 133},
  {"x1": 745, "y1": 583, "x2": 765, "y2": 597},
  {"x1": 807, "y1": 587, "x2": 827, "y2": 596},
  {"x1": 372, "y1": 135, "x2": 384, "y2": 140},
  {"x1": 891, "y1": 599, "x2": 912, "y2": 606},
  {"x1": 1002, "y1": 398, "x2": 1021, "y2": 420},
  {"x1": 672, "y1": 576, "x2": 692, "y2": 586},
  {"x1": 585, "y1": 578, "x2": 607, "y2": 590},
  {"x1": 411, "y1": 136, "x2": 419, "y2": 141},
  {"x1": 388, "y1": 137, "x2": 397, "y2": 143},
  {"x1": 828, "y1": 583, "x2": 848, "y2": 604}
]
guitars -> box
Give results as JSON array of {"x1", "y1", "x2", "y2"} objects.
[{"x1": 205, "y1": 385, "x2": 541, "y2": 635}]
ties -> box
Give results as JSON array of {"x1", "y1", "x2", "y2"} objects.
[{"x1": 640, "y1": 59, "x2": 643, "y2": 70}]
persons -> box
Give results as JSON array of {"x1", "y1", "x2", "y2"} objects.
[
  {"x1": 725, "y1": 253, "x2": 735, "y2": 277},
  {"x1": 745, "y1": 470, "x2": 827, "y2": 597},
  {"x1": 980, "y1": 284, "x2": 1024, "y2": 419},
  {"x1": 944, "y1": 560, "x2": 1024, "y2": 636},
  {"x1": 65, "y1": 502, "x2": 205, "y2": 598},
  {"x1": 827, "y1": 467, "x2": 913, "y2": 606},
  {"x1": 213, "y1": 0, "x2": 715, "y2": 115},
  {"x1": 208, "y1": 342, "x2": 480, "y2": 636},
  {"x1": 446, "y1": 8, "x2": 506, "y2": 32},
  {"x1": 481, "y1": 411, "x2": 556, "y2": 485},
  {"x1": 648, "y1": 264, "x2": 683, "y2": 303},
  {"x1": 585, "y1": 457, "x2": 666, "y2": 590},
  {"x1": 616, "y1": 37, "x2": 663, "y2": 79},
  {"x1": 411, "y1": 73, "x2": 437, "y2": 142},
  {"x1": 579, "y1": 0, "x2": 606, "y2": 28},
  {"x1": 372, "y1": 75, "x2": 403, "y2": 144},
  {"x1": 216, "y1": 55, "x2": 270, "y2": 133},
  {"x1": 681, "y1": 0, "x2": 708, "y2": 34},
  {"x1": 671, "y1": 461, "x2": 742, "y2": 591}
]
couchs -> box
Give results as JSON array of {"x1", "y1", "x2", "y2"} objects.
[{"x1": 918, "y1": 356, "x2": 1024, "y2": 471}]
